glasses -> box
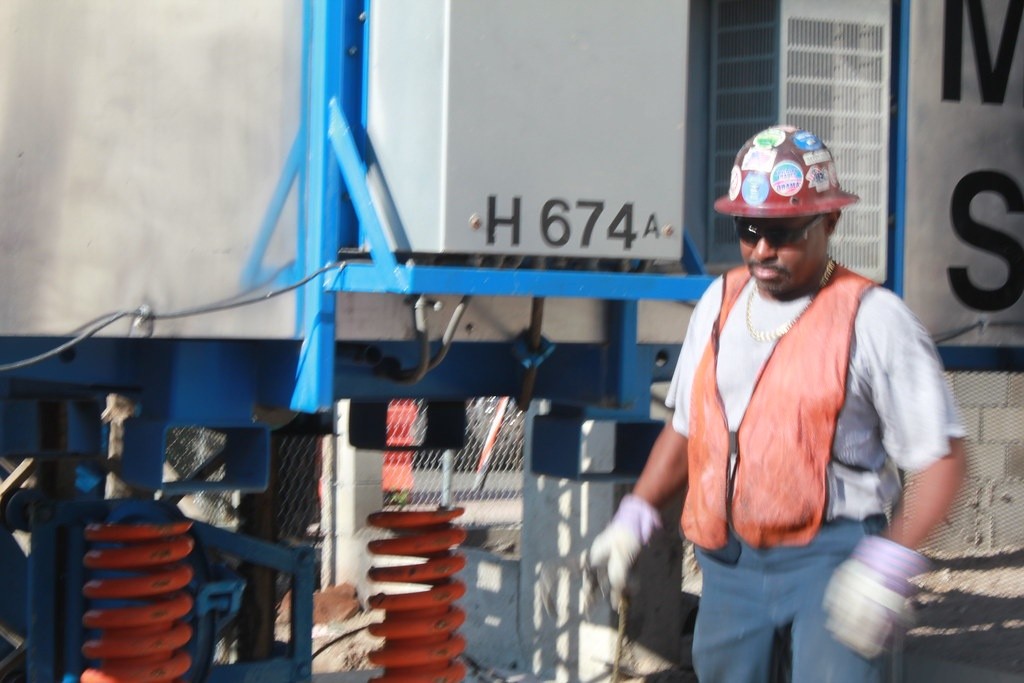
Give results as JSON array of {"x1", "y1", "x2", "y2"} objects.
[{"x1": 733, "y1": 213, "x2": 826, "y2": 246}]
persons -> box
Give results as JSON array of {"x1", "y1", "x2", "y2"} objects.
[{"x1": 576, "y1": 119, "x2": 971, "y2": 683}]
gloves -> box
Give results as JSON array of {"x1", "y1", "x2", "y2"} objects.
[
  {"x1": 578, "y1": 492, "x2": 664, "y2": 613},
  {"x1": 822, "y1": 535, "x2": 931, "y2": 662}
]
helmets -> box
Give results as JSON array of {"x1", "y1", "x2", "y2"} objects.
[{"x1": 713, "y1": 123, "x2": 860, "y2": 219}]
